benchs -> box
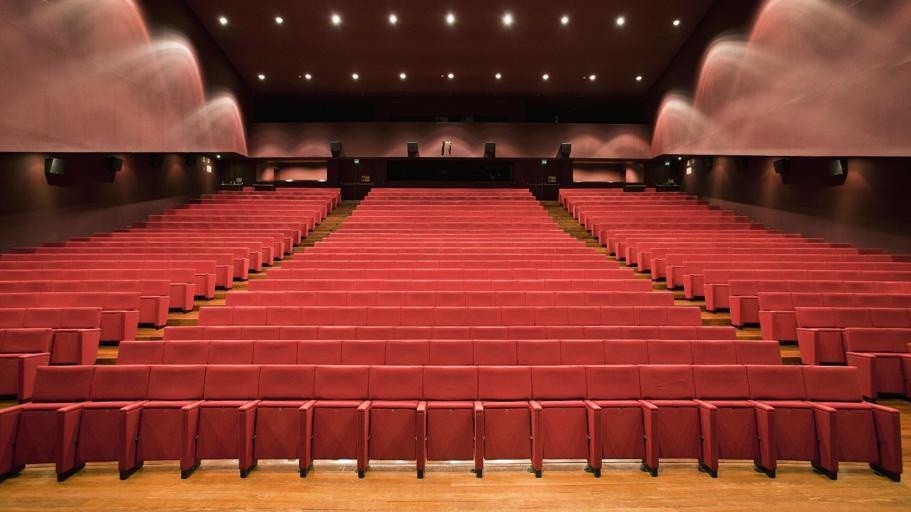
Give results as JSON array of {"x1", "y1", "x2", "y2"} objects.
[{"x1": 0, "y1": 187, "x2": 911, "y2": 482}]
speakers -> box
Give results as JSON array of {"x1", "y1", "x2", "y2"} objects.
[
  {"x1": 44, "y1": 157, "x2": 67, "y2": 176},
  {"x1": 106, "y1": 157, "x2": 123, "y2": 171},
  {"x1": 408, "y1": 142, "x2": 418, "y2": 153},
  {"x1": 831, "y1": 158, "x2": 848, "y2": 177},
  {"x1": 702, "y1": 158, "x2": 713, "y2": 168},
  {"x1": 561, "y1": 143, "x2": 571, "y2": 153},
  {"x1": 486, "y1": 143, "x2": 495, "y2": 153},
  {"x1": 149, "y1": 153, "x2": 163, "y2": 166},
  {"x1": 773, "y1": 158, "x2": 789, "y2": 174},
  {"x1": 734, "y1": 158, "x2": 747, "y2": 171},
  {"x1": 331, "y1": 143, "x2": 341, "y2": 152},
  {"x1": 185, "y1": 152, "x2": 197, "y2": 166}
]
